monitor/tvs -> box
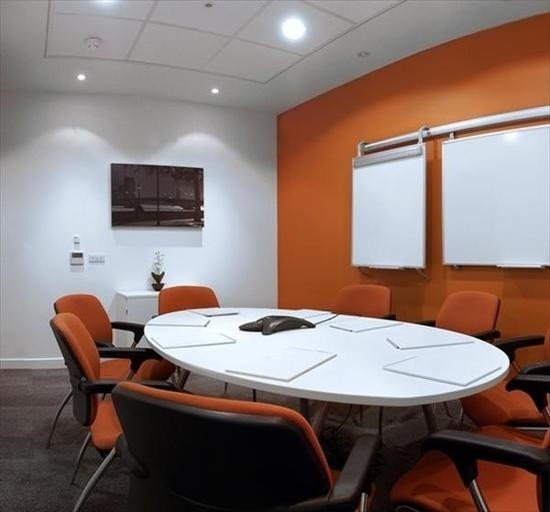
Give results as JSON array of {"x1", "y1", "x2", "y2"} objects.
[{"x1": 111, "y1": 163, "x2": 204, "y2": 228}]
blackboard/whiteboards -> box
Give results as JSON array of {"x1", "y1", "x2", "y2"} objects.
[
  {"x1": 441, "y1": 124, "x2": 550, "y2": 266},
  {"x1": 349, "y1": 142, "x2": 427, "y2": 269}
]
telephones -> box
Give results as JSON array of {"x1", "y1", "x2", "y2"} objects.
[{"x1": 238, "y1": 315, "x2": 315, "y2": 335}]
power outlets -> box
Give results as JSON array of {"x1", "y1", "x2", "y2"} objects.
[{"x1": 89, "y1": 254, "x2": 105, "y2": 263}]
